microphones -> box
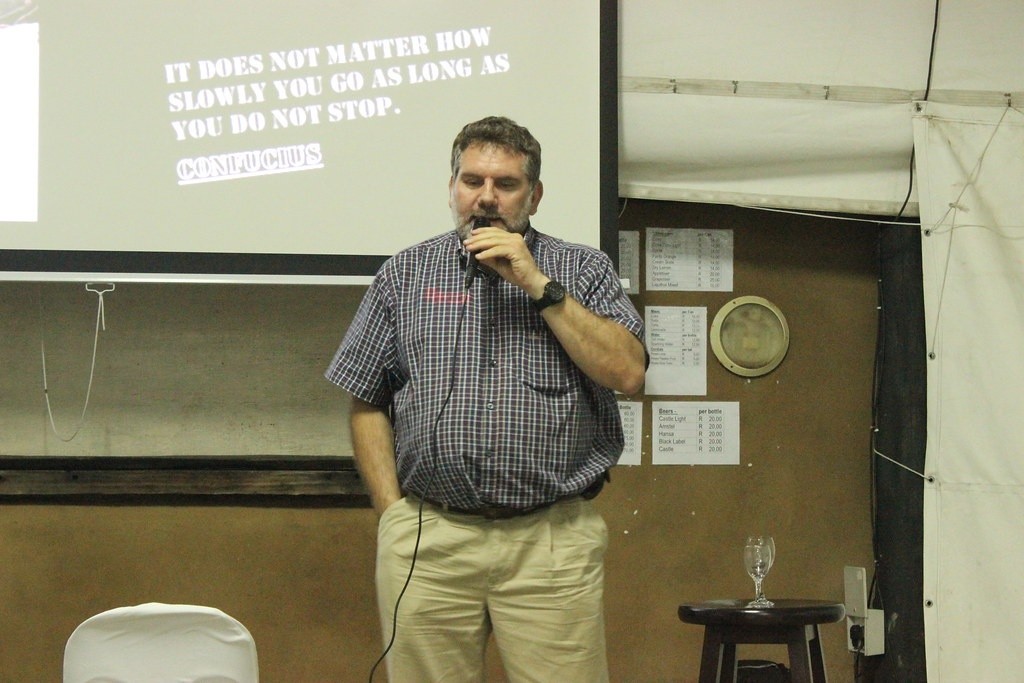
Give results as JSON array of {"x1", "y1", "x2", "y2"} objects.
[{"x1": 464, "y1": 217, "x2": 491, "y2": 288}]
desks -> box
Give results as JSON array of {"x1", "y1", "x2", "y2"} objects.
[{"x1": 678, "y1": 599, "x2": 846, "y2": 683}]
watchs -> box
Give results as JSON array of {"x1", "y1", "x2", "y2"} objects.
[{"x1": 529, "y1": 278, "x2": 567, "y2": 312}]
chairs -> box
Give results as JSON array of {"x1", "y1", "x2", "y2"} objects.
[{"x1": 63, "y1": 602, "x2": 260, "y2": 683}]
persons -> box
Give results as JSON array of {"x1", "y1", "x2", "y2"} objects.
[{"x1": 323, "y1": 115, "x2": 650, "y2": 683}]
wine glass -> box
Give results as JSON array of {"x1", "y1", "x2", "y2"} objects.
[
  {"x1": 744, "y1": 545, "x2": 773, "y2": 607},
  {"x1": 746, "y1": 536, "x2": 775, "y2": 606}
]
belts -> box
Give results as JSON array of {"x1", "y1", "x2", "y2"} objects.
[{"x1": 406, "y1": 488, "x2": 568, "y2": 520}]
